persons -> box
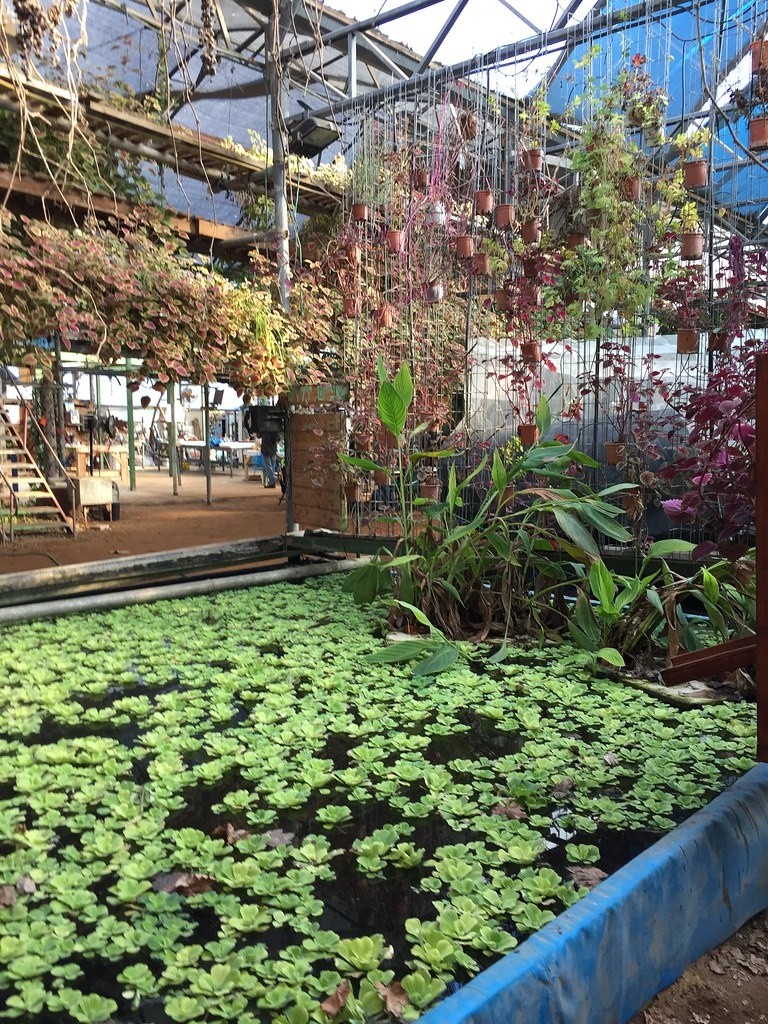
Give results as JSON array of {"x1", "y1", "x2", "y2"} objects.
[{"x1": 258, "y1": 432, "x2": 280, "y2": 488}]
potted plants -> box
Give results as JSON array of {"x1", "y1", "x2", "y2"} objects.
[{"x1": 342, "y1": 39, "x2": 768, "y2": 512}]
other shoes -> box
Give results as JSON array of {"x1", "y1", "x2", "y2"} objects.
[{"x1": 264, "y1": 484, "x2": 276, "y2": 488}]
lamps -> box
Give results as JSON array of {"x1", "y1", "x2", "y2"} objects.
[
  {"x1": 103, "y1": 415, "x2": 116, "y2": 439},
  {"x1": 284, "y1": 116, "x2": 346, "y2": 161},
  {"x1": 245, "y1": 404, "x2": 293, "y2": 434}
]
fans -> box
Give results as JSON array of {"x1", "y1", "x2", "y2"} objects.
[{"x1": 83, "y1": 404, "x2": 110, "y2": 476}]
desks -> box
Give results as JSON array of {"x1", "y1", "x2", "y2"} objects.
[
  {"x1": 157, "y1": 439, "x2": 254, "y2": 478},
  {"x1": 77, "y1": 443, "x2": 129, "y2": 474}
]
